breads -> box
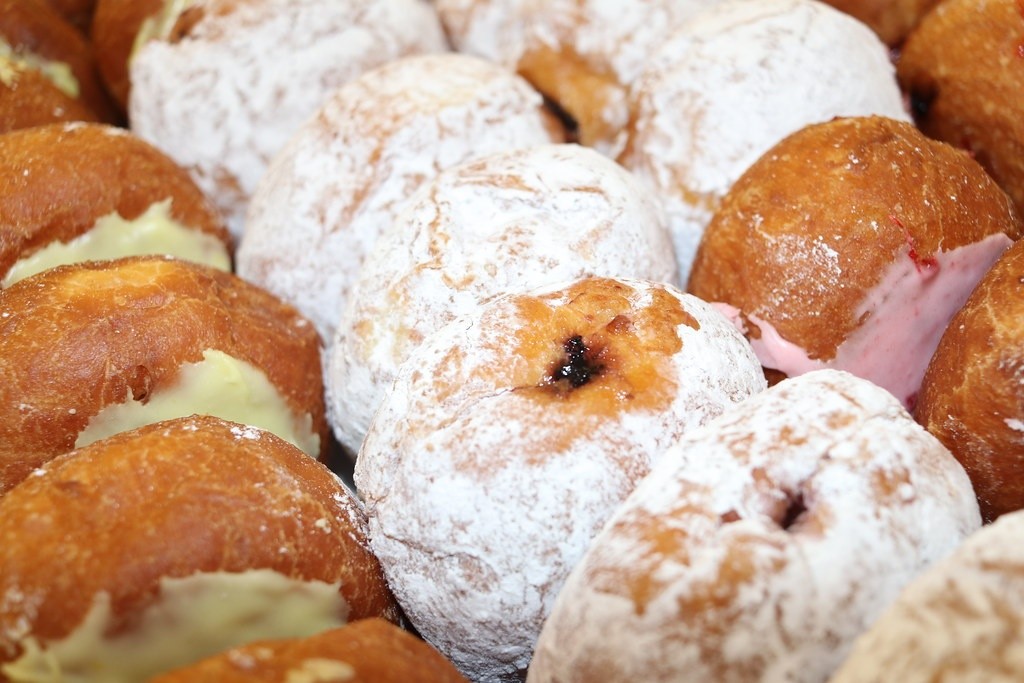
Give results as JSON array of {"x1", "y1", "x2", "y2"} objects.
[{"x1": 0, "y1": 0, "x2": 1024, "y2": 682}]
